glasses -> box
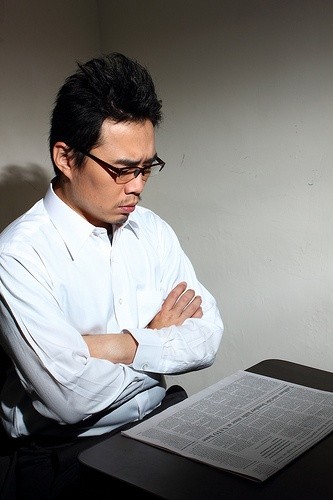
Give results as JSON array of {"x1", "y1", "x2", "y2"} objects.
[{"x1": 81, "y1": 150, "x2": 166, "y2": 181}]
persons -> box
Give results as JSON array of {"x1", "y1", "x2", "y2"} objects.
[{"x1": 0, "y1": 53, "x2": 225, "y2": 498}]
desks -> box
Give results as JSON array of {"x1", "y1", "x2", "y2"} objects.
[{"x1": 78, "y1": 358, "x2": 333, "y2": 500}]
